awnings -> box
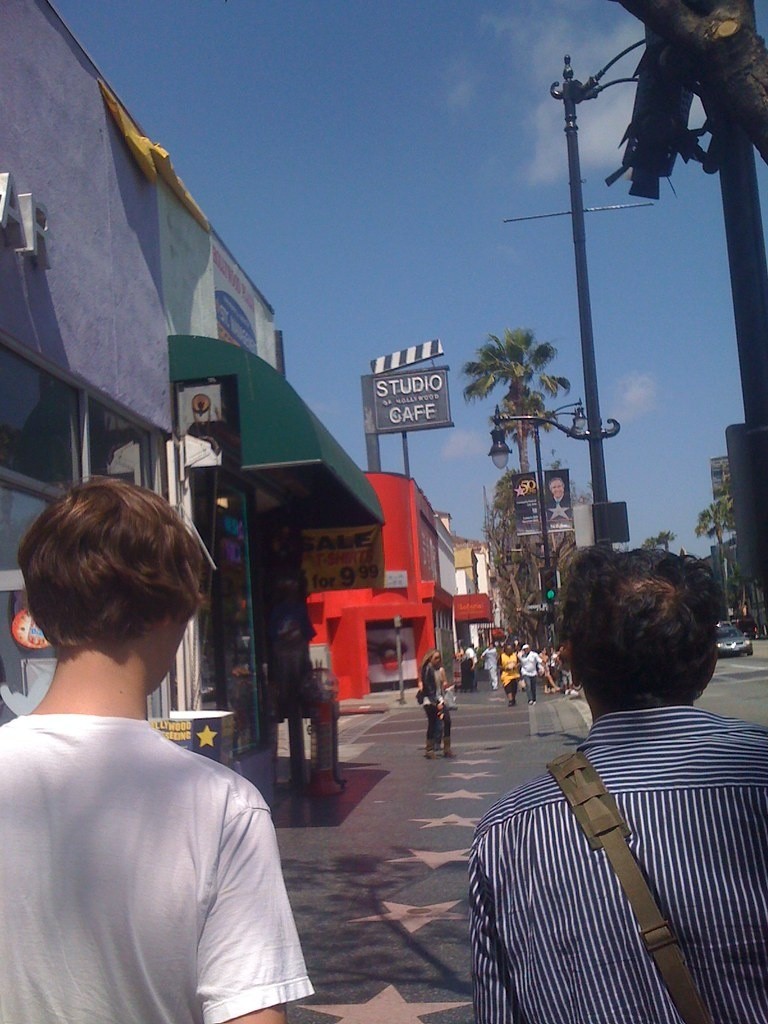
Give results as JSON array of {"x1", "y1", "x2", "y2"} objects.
[{"x1": 169, "y1": 334, "x2": 387, "y2": 528}]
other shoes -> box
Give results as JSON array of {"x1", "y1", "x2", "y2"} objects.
[
  {"x1": 533, "y1": 701, "x2": 536, "y2": 705},
  {"x1": 529, "y1": 700, "x2": 533, "y2": 704},
  {"x1": 509, "y1": 700, "x2": 514, "y2": 706}
]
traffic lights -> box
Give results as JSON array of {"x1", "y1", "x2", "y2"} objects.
[
  {"x1": 538, "y1": 566, "x2": 557, "y2": 604},
  {"x1": 715, "y1": 626, "x2": 754, "y2": 659}
]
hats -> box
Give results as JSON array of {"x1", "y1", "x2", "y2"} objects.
[{"x1": 522, "y1": 645, "x2": 529, "y2": 649}]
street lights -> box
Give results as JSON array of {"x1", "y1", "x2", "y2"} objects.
[{"x1": 532, "y1": 398, "x2": 589, "y2": 629}]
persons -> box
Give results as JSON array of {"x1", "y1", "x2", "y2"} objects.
[
  {"x1": 415, "y1": 648, "x2": 460, "y2": 760},
  {"x1": 468, "y1": 541, "x2": 765, "y2": 1024},
  {"x1": 0, "y1": 475, "x2": 316, "y2": 1024},
  {"x1": 458, "y1": 637, "x2": 584, "y2": 706}
]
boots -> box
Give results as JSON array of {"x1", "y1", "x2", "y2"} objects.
[
  {"x1": 443, "y1": 736, "x2": 457, "y2": 756},
  {"x1": 425, "y1": 739, "x2": 441, "y2": 759}
]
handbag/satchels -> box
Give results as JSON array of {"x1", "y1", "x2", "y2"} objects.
[{"x1": 444, "y1": 684, "x2": 458, "y2": 710}]
